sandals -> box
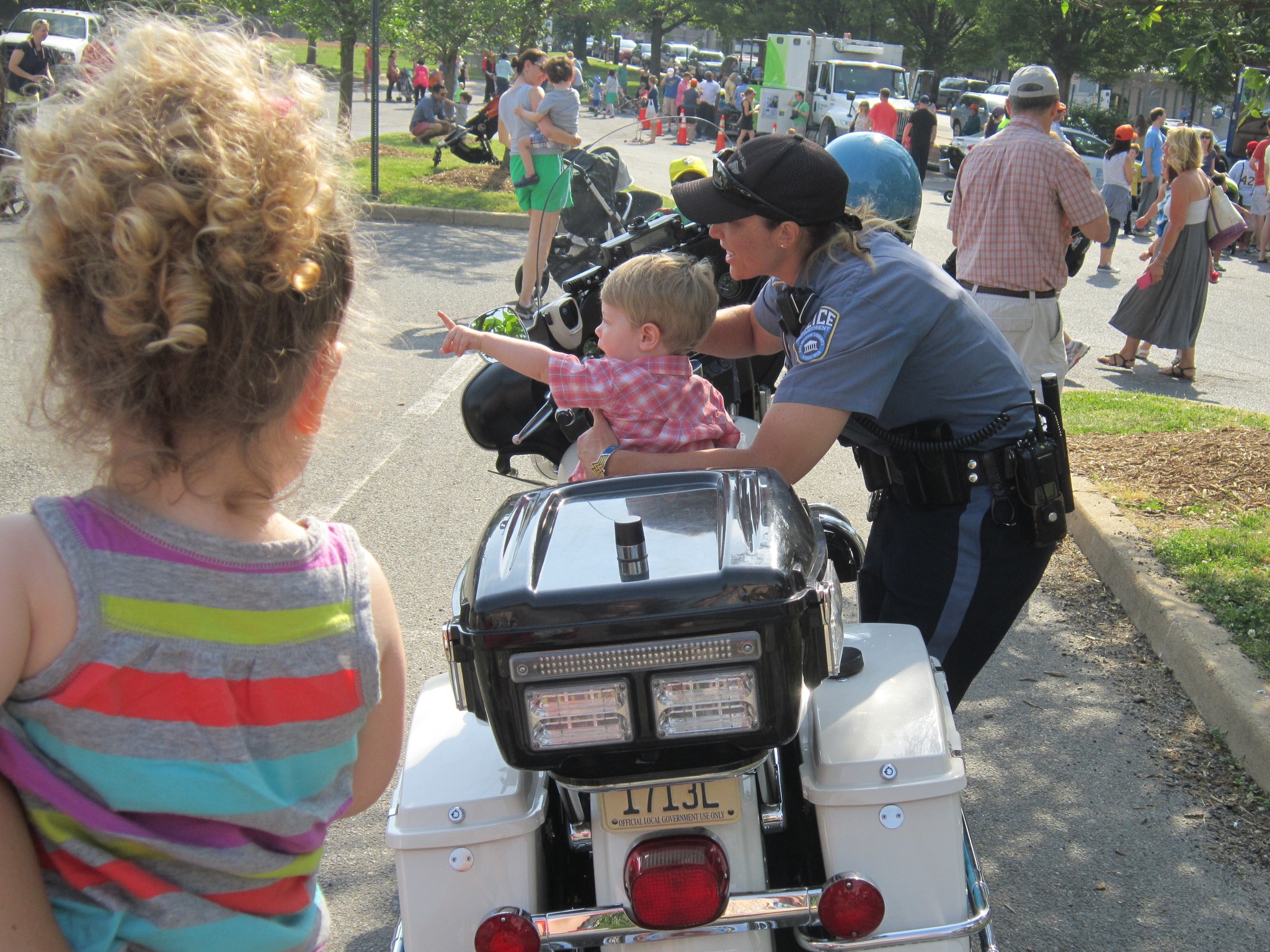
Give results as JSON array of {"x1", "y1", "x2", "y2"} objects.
[
  {"x1": 1135, "y1": 346, "x2": 1150, "y2": 359},
  {"x1": 1158, "y1": 365, "x2": 1196, "y2": 380},
  {"x1": 1096, "y1": 353, "x2": 1136, "y2": 369},
  {"x1": 513, "y1": 172, "x2": 539, "y2": 188},
  {"x1": 1171, "y1": 349, "x2": 1182, "y2": 366}
]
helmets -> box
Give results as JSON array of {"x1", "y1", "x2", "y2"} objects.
[{"x1": 824, "y1": 131, "x2": 922, "y2": 246}]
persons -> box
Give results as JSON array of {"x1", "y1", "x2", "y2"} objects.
[
  {"x1": 438, "y1": 250, "x2": 741, "y2": 482},
  {"x1": 1097, "y1": 124, "x2": 1138, "y2": 273},
  {"x1": 947, "y1": 64, "x2": 1112, "y2": 407},
  {"x1": 1061, "y1": 329, "x2": 1090, "y2": 371},
  {"x1": 364, "y1": 47, "x2": 995, "y2": 169},
  {"x1": 1096, "y1": 126, "x2": 1211, "y2": 380},
  {"x1": 0, "y1": 775, "x2": 68, "y2": 952},
  {"x1": 0, "y1": 8, "x2": 402, "y2": 952},
  {"x1": 1135, "y1": 251, "x2": 1183, "y2": 366},
  {"x1": 1050, "y1": 102, "x2": 1072, "y2": 145},
  {"x1": 983, "y1": 106, "x2": 1005, "y2": 138},
  {"x1": 1124, "y1": 143, "x2": 1148, "y2": 235},
  {"x1": 1179, "y1": 105, "x2": 1189, "y2": 120},
  {"x1": 7, "y1": 18, "x2": 54, "y2": 106},
  {"x1": 1201, "y1": 117, "x2": 1270, "y2": 272},
  {"x1": 1136, "y1": 157, "x2": 1220, "y2": 284},
  {"x1": 498, "y1": 49, "x2": 575, "y2": 320},
  {"x1": 78, "y1": 22, "x2": 118, "y2": 81},
  {"x1": 996, "y1": 105, "x2": 1011, "y2": 133},
  {"x1": 1133, "y1": 108, "x2": 1184, "y2": 236},
  {"x1": 578, "y1": 133, "x2": 1056, "y2": 714},
  {"x1": 513, "y1": 56, "x2": 580, "y2": 188}
]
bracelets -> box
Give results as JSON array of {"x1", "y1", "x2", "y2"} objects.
[
  {"x1": 1154, "y1": 251, "x2": 1168, "y2": 270},
  {"x1": 590, "y1": 445, "x2": 625, "y2": 478}
]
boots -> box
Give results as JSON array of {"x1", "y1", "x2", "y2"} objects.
[{"x1": 1238, "y1": 241, "x2": 1250, "y2": 251}]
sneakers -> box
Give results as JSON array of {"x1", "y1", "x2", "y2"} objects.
[
  {"x1": 514, "y1": 299, "x2": 550, "y2": 320},
  {"x1": 1241, "y1": 247, "x2": 1267, "y2": 256},
  {"x1": 1065, "y1": 339, "x2": 1091, "y2": 371},
  {"x1": 1133, "y1": 230, "x2": 1153, "y2": 236},
  {"x1": 1146, "y1": 227, "x2": 1157, "y2": 236},
  {"x1": 1097, "y1": 262, "x2": 1121, "y2": 273}
]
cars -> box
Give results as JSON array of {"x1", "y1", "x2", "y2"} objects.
[
  {"x1": 953, "y1": 126, "x2": 1144, "y2": 194},
  {"x1": 1159, "y1": 118, "x2": 1247, "y2": 173},
  {"x1": 585, "y1": 33, "x2": 759, "y2": 87}
]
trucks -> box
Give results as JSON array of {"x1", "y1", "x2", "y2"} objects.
[{"x1": 745, "y1": 28, "x2": 934, "y2": 150}]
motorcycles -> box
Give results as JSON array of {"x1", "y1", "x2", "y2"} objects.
[
  {"x1": 383, "y1": 118, "x2": 1001, "y2": 952},
  {"x1": 0, "y1": 147, "x2": 30, "y2": 222}
]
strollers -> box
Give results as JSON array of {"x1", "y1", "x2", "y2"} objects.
[{"x1": 433, "y1": 94, "x2": 503, "y2": 166}]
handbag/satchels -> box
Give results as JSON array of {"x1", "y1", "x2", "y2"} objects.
[{"x1": 1198, "y1": 168, "x2": 1248, "y2": 251}]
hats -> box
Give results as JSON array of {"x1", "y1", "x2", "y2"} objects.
[
  {"x1": 1115, "y1": 124, "x2": 1138, "y2": 140},
  {"x1": 670, "y1": 133, "x2": 850, "y2": 227},
  {"x1": 1247, "y1": 141, "x2": 1259, "y2": 151},
  {"x1": 1009, "y1": 64, "x2": 1059, "y2": 98},
  {"x1": 669, "y1": 155, "x2": 708, "y2": 182}
]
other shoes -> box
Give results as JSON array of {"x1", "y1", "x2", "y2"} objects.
[
  {"x1": 412, "y1": 137, "x2": 422, "y2": 146},
  {"x1": 422, "y1": 137, "x2": 432, "y2": 146},
  {"x1": 1257, "y1": 257, "x2": 1268, "y2": 263}
]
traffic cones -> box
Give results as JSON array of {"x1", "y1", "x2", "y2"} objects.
[
  {"x1": 638, "y1": 90, "x2": 652, "y2": 131},
  {"x1": 672, "y1": 109, "x2": 691, "y2": 145},
  {"x1": 656, "y1": 116, "x2": 664, "y2": 136},
  {"x1": 712, "y1": 115, "x2": 726, "y2": 154}
]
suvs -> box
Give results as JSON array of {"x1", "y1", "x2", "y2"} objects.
[
  {"x1": 934, "y1": 76, "x2": 990, "y2": 115},
  {"x1": 950, "y1": 81, "x2": 1011, "y2": 136},
  {"x1": 0, "y1": 8, "x2": 106, "y2": 86}
]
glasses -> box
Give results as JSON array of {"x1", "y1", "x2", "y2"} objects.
[
  {"x1": 1200, "y1": 135, "x2": 1211, "y2": 139},
  {"x1": 533, "y1": 61, "x2": 547, "y2": 73},
  {"x1": 671, "y1": 177, "x2": 707, "y2": 188},
  {"x1": 438, "y1": 91, "x2": 448, "y2": 96}
]
